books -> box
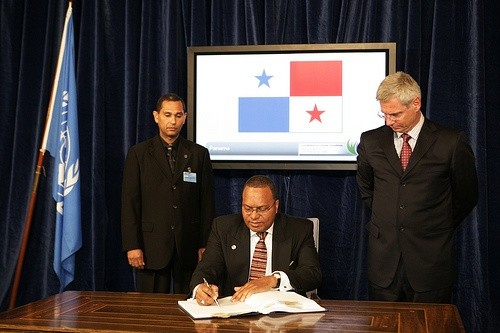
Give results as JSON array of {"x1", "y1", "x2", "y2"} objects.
[{"x1": 176, "y1": 290, "x2": 325, "y2": 319}]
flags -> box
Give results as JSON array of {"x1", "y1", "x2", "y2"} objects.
[{"x1": 39, "y1": 3, "x2": 83, "y2": 295}]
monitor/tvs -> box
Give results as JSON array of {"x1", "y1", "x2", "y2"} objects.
[{"x1": 186, "y1": 42, "x2": 396, "y2": 171}]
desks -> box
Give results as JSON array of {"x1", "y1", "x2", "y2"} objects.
[{"x1": 0, "y1": 289, "x2": 468, "y2": 333}]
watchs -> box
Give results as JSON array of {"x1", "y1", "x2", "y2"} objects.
[{"x1": 272, "y1": 272, "x2": 281, "y2": 289}]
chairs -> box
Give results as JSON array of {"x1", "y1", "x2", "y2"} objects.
[{"x1": 305, "y1": 216, "x2": 322, "y2": 297}]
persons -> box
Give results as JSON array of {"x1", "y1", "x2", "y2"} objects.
[
  {"x1": 188, "y1": 174, "x2": 322, "y2": 310},
  {"x1": 357, "y1": 71, "x2": 476, "y2": 303},
  {"x1": 118, "y1": 92, "x2": 216, "y2": 300}
]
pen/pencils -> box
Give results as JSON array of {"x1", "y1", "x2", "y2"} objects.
[{"x1": 203, "y1": 278, "x2": 221, "y2": 309}]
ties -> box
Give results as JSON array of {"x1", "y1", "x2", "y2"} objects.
[
  {"x1": 248, "y1": 232, "x2": 269, "y2": 282},
  {"x1": 400, "y1": 134, "x2": 412, "y2": 171},
  {"x1": 167, "y1": 145, "x2": 174, "y2": 175}
]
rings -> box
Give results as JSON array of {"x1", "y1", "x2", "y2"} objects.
[{"x1": 201, "y1": 299, "x2": 206, "y2": 304}]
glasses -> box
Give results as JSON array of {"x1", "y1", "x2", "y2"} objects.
[
  {"x1": 242, "y1": 202, "x2": 275, "y2": 214},
  {"x1": 378, "y1": 103, "x2": 410, "y2": 120}
]
